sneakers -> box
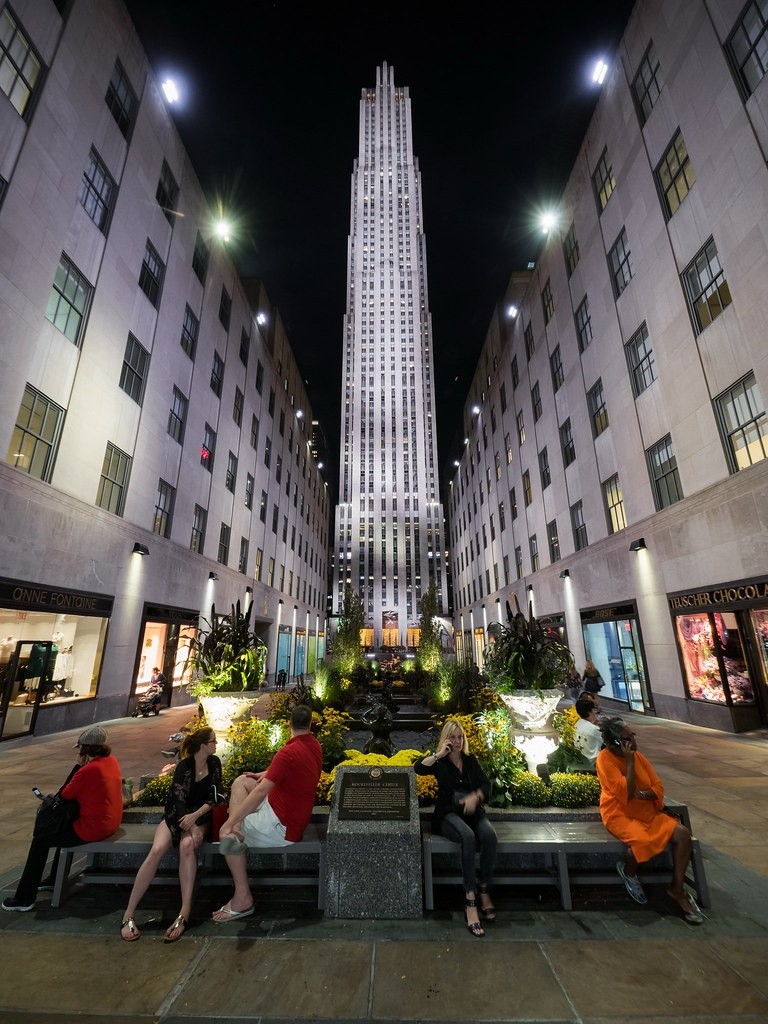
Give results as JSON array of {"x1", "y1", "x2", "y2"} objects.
[
  {"x1": 38, "y1": 875, "x2": 56, "y2": 890},
  {"x1": 616, "y1": 862, "x2": 647, "y2": 903},
  {"x1": 2, "y1": 897, "x2": 35, "y2": 911},
  {"x1": 668, "y1": 886, "x2": 703, "y2": 923}
]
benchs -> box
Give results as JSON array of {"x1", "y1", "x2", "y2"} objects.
[
  {"x1": 50, "y1": 823, "x2": 325, "y2": 910},
  {"x1": 421, "y1": 821, "x2": 711, "y2": 911}
]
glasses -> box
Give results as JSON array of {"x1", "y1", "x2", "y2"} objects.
[
  {"x1": 621, "y1": 733, "x2": 636, "y2": 741},
  {"x1": 201, "y1": 738, "x2": 216, "y2": 745}
]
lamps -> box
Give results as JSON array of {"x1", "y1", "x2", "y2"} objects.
[
  {"x1": 132, "y1": 542, "x2": 150, "y2": 555},
  {"x1": 558, "y1": 569, "x2": 570, "y2": 580},
  {"x1": 451, "y1": 584, "x2": 533, "y2": 620},
  {"x1": 208, "y1": 571, "x2": 220, "y2": 579},
  {"x1": 278, "y1": 599, "x2": 328, "y2": 620},
  {"x1": 630, "y1": 538, "x2": 646, "y2": 551},
  {"x1": 247, "y1": 586, "x2": 253, "y2": 592}
]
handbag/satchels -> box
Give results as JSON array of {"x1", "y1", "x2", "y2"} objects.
[
  {"x1": 597, "y1": 677, "x2": 605, "y2": 687},
  {"x1": 33, "y1": 793, "x2": 79, "y2": 837}
]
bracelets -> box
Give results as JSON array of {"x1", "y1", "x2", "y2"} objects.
[
  {"x1": 432, "y1": 752, "x2": 439, "y2": 762},
  {"x1": 197, "y1": 810, "x2": 203, "y2": 815},
  {"x1": 476, "y1": 791, "x2": 481, "y2": 803}
]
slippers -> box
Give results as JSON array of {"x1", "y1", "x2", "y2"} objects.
[
  {"x1": 163, "y1": 915, "x2": 189, "y2": 940},
  {"x1": 219, "y1": 836, "x2": 247, "y2": 854},
  {"x1": 213, "y1": 905, "x2": 254, "y2": 921},
  {"x1": 120, "y1": 917, "x2": 140, "y2": 941}
]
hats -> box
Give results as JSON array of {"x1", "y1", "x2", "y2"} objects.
[
  {"x1": 153, "y1": 667, "x2": 160, "y2": 671},
  {"x1": 71, "y1": 726, "x2": 108, "y2": 748}
]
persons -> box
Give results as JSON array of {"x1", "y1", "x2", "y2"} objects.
[
  {"x1": 573, "y1": 692, "x2": 604, "y2": 766},
  {"x1": 275, "y1": 670, "x2": 282, "y2": 690},
  {"x1": 212, "y1": 706, "x2": 322, "y2": 923},
  {"x1": 596, "y1": 719, "x2": 702, "y2": 923},
  {"x1": 265, "y1": 669, "x2": 270, "y2": 685},
  {"x1": 281, "y1": 669, "x2": 287, "y2": 691},
  {"x1": 120, "y1": 727, "x2": 225, "y2": 941},
  {"x1": 2, "y1": 725, "x2": 123, "y2": 911},
  {"x1": 151, "y1": 667, "x2": 167, "y2": 715},
  {"x1": 582, "y1": 661, "x2": 602, "y2": 696},
  {"x1": 415, "y1": 720, "x2": 498, "y2": 936}
]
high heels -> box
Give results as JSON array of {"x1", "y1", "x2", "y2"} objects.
[
  {"x1": 464, "y1": 899, "x2": 484, "y2": 936},
  {"x1": 477, "y1": 885, "x2": 495, "y2": 920}
]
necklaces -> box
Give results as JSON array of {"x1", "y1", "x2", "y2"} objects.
[{"x1": 195, "y1": 764, "x2": 208, "y2": 776}]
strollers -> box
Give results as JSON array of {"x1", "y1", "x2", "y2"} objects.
[{"x1": 131, "y1": 684, "x2": 164, "y2": 718}]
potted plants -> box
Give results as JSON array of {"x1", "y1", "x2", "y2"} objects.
[
  {"x1": 178, "y1": 601, "x2": 269, "y2": 735},
  {"x1": 482, "y1": 596, "x2": 580, "y2": 734}
]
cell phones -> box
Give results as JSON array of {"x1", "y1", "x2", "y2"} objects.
[
  {"x1": 32, "y1": 788, "x2": 45, "y2": 800},
  {"x1": 446, "y1": 744, "x2": 451, "y2": 751},
  {"x1": 611, "y1": 737, "x2": 633, "y2": 750}
]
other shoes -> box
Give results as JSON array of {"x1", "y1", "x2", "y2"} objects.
[{"x1": 161, "y1": 748, "x2": 178, "y2": 758}]
